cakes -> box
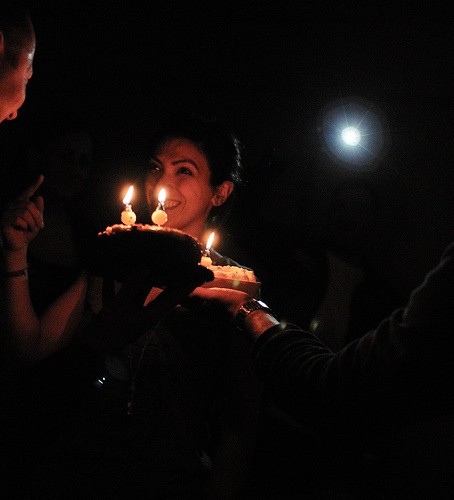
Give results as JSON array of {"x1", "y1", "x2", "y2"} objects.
[
  {"x1": 196, "y1": 264, "x2": 262, "y2": 295},
  {"x1": 94, "y1": 205, "x2": 203, "y2": 279}
]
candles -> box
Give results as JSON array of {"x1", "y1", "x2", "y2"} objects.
[
  {"x1": 201, "y1": 231, "x2": 216, "y2": 267},
  {"x1": 151, "y1": 187, "x2": 168, "y2": 227},
  {"x1": 121, "y1": 185, "x2": 137, "y2": 225}
]
